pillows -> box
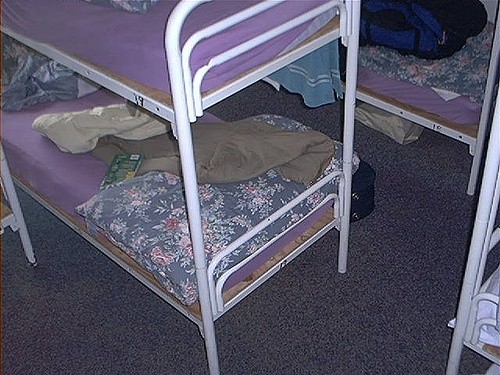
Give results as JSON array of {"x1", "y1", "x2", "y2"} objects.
[{"x1": 74, "y1": 113, "x2": 359, "y2": 306}]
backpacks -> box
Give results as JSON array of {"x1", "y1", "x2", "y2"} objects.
[{"x1": 359, "y1": 0, "x2": 487, "y2": 59}]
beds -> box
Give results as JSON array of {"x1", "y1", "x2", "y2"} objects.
[
  {"x1": 0, "y1": 0, "x2": 361, "y2": 375},
  {"x1": 338, "y1": 0, "x2": 500, "y2": 197}
]
